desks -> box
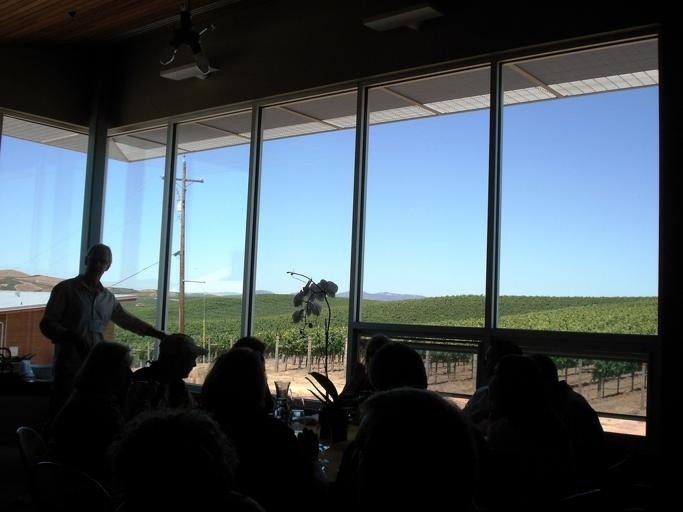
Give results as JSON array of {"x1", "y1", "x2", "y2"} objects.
[{"x1": 305, "y1": 423, "x2": 360, "y2": 486}]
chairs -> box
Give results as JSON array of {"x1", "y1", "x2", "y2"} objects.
[{"x1": 14, "y1": 425, "x2": 115, "y2": 512}]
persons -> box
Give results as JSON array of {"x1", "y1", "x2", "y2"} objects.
[
  {"x1": 39, "y1": 245, "x2": 169, "y2": 418},
  {"x1": 40, "y1": 331, "x2": 319, "y2": 511},
  {"x1": 338, "y1": 334, "x2": 620, "y2": 510}
]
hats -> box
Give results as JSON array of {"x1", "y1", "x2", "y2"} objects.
[
  {"x1": 366, "y1": 333, "x2": 427, "y2": 389},
  {"x1": 160, "y1": 334, "x2": 209, "y2": 354}
]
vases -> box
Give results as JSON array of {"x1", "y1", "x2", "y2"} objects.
[{"x1": 317, "y1": 407, "x2": 348, "y2": 441}]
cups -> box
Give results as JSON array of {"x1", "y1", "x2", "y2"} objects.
[{"x1": 274, "y1": 381, "x2": 290, "y2": 398}]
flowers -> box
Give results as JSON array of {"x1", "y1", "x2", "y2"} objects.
[{"x1": 287, "y1": 269, "x2": 343, "y2": 411}]
